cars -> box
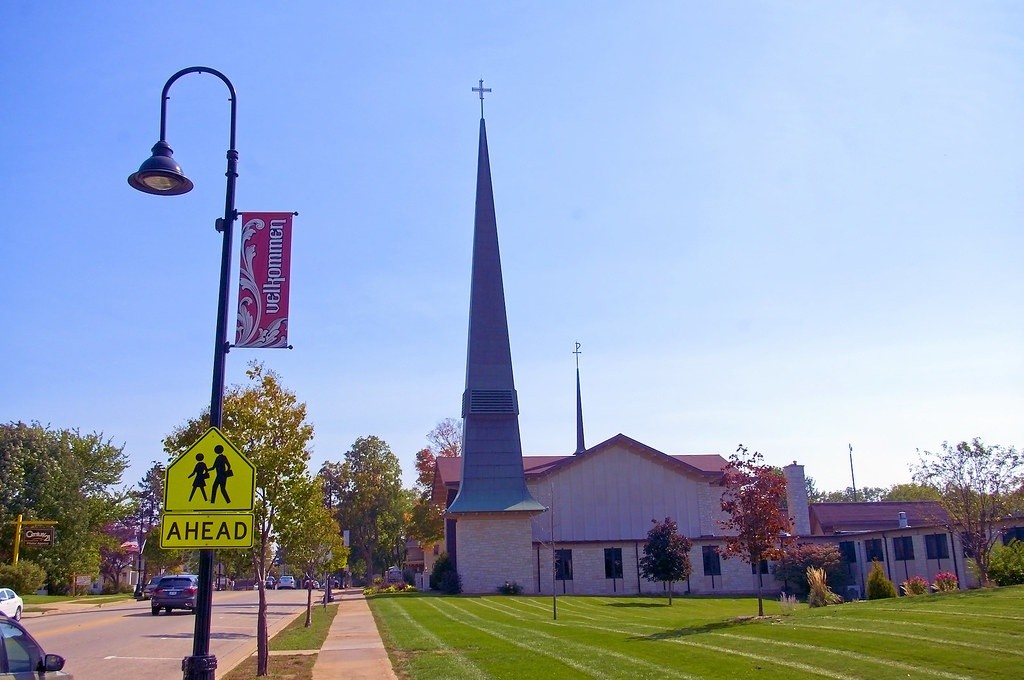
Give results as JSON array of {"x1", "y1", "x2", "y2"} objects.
[
  {"x1": 263, "y1": 576, "x2": 276, "y2": 589},
  {"x1": 304, "y1": 580, "x2": 318, "y2": 589},
  {"x1": 150, "y1": 572, "x2": 198, "y2": 616},
  {"x1": 276, "y1": 575, "x2": 295, "y2": 589},
  {"x1": 145, "y1": 577, "x2": 163, "y2": 598},
  {"x1": 216, "y1": 576, "x2": 230, "y2": 591},
  {"x1": 1, "y1": 586, "x2": 26, "y2": 621},
  {"x1": 0, "y1": 609, "x2": 74, "y2": 680}
]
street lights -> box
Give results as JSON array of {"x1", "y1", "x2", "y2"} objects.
[{"x1": 128, "y1": 66, "x2": 241, "y2": 680}]
